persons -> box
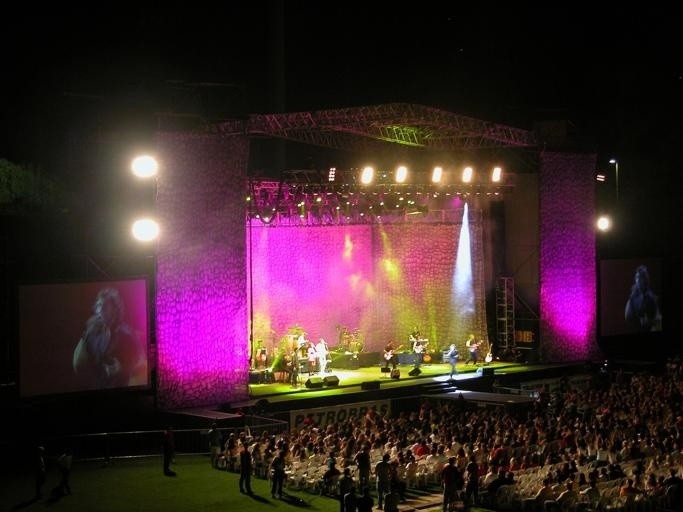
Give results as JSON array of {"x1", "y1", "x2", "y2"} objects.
[
  {"x1": 255, "y1": 336, "x2": 330, "y2": 387},
  {"x1": 622, "y1": 263, "x2": 662, "y2": 334},
  {"x1": 413, "y1": 341, "x2": 429, "y2": 369},
  {"x1": 31, "y1": 445, "x2": 47, "y2": 499},
  {"x1": 464, "y1": 338, "x2": 482, "y2": 364},
  {"x1": 71, "y1": 286, "x2": 146, "y2": 390},
  {"x1": 206, "y1": 361, "x2": 681, "y2": 511},
  {"x1": 383, "y1": 340, "x2": 401, "y2": 370},
  {"x1": 158, "y1": 424, "x2": 177, "y2": 477},
  {"x1": 53, "y1": 444, "x2": 74, "y2": 496},
  {"x1": 446, "y1": 343, "x2": 459, "y2": 378}
]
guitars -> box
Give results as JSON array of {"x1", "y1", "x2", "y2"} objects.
[
  {"x1": 485, "y1": 344, "x2": 493, "y2": 362},
  {"x1": 384, "y1": 345, "x2": 402, "y2": 360},
  {"x1": 469, "y1": 341, "x2": 482, "y2": 352},
  {"x1": 415, "y1": 343, "x2": 428, "y2": 354}
]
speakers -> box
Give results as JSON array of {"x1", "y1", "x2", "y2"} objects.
[
  {"x1": 361, "y1": 380, "x2": 380, "y2": 390},
  {"x1": 323, "y1": 375, "x2": 339, "y2": 385},
  {"x1": 477, "y1": 367, "x2": 494, "y2": 376},
  {"x1": 305, "y1": 377, "x2": 323, "y2": 388},
  {"x1": 391, "y1": 369, "x2": 400, "y2": 379},
  {"x1": 407, "y1": 368, "x2": 422, "y2": 376}
]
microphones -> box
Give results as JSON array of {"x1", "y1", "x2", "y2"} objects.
[{"x1": 84, "y1": 312, "x2": 104, "y2": 343}]
